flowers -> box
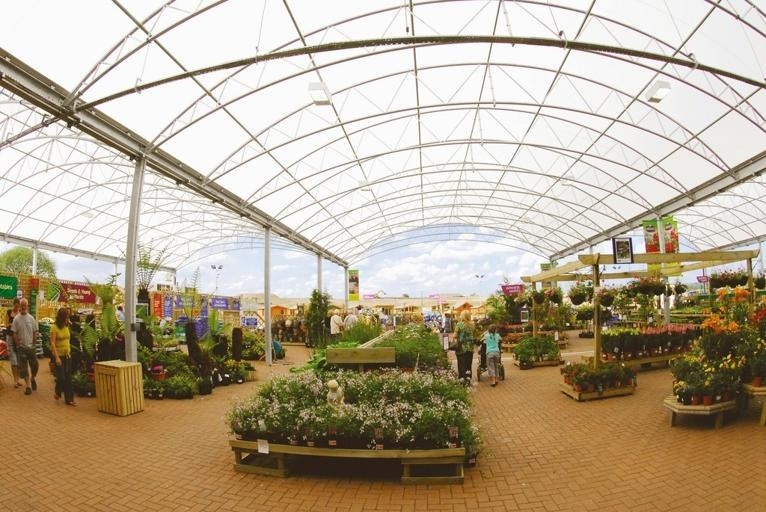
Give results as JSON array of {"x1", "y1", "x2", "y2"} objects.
[
  {"x1": 492, "y1": 268, "x2": 766, "y2": 429},
  {"x1": 224, "y1": 267, "x2": 489, "y2": 456}
]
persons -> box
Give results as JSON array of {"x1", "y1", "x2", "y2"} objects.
[
  {"x1": 476, "y1": 340, "x2": 504, "y2": 355},
  {"x1": 452, "y1": 309, "x2": 476, "y2": 388},
  {"x1": 50, "y1": 306, "x2": 78, "y2": 406},
  {"x1": 10, "y1": 295, "x2": 40, "y2": 396},
  {"x1": 66, "y1": 307, "x2": 95, "y2": 347},
  {"x1": 343, "y1": 310, "x2": 358, "y2": 329},
  {"x1": 329, "y1": 307, "x2": 344, "y2": 345},
  {"x1": 320, "y1": 309, "x2": 334, "y2": 346},
  {"x1": 115, "y1": 305, "x2": 125, "y2": 320},
  {"x1": 4, "y1": 298, "x2": 24, "y2": 389},
  {"x1": 327, "y1": 380, "x2": 345, "y2": 411},
  {"x1": 480, "y1": 325, "x2": 504, "y2": 387}
]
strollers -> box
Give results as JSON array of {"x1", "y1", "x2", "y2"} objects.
[{"x1": 477, "y1": 340, "x2": 505, "y2": 382}]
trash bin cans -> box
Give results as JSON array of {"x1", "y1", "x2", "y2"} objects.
[{"x1": 92, "y1": 360, "x2": 145, "y2": 416}]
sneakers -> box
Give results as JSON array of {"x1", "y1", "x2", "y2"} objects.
[
  {"x1": 17, "y1": 382, "x2": 22, "y2": 386},
  {"x1": 14, "y1": 384, "x2": 18, "y2": 388},
  {"x1": 24, "y1": 386, "x2": 32, "y2": 395},
  {"x1": 30, "y1": 378, "x2": 37, "y2": 391}
]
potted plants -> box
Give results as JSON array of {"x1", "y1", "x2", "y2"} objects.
[{"x1": 44, "y1": 237, "x2": 230, "y2": 399}]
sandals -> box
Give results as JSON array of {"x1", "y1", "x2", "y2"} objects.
[
  {"x1": 54, "y1": 391, "x2": 59, "y2": 400},
  {"x1": 66, "y1": 402, "x2": 76, "y2": 407}
]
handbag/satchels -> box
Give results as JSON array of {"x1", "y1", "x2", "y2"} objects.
[{"x1": 448, "y1": 338, "x2": 461, "y2": 351}]
